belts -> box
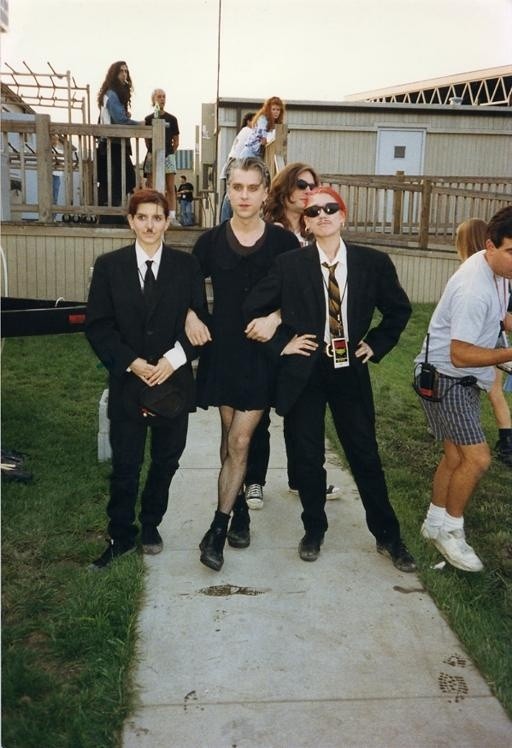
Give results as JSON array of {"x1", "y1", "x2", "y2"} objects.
[{"x1": 318, "y1": 344, "x2": 358, "y2": 359}]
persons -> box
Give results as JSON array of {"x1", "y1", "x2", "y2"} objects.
[
  {"x1": 414, "y1": 203, "x2": 511, "y2": 574},
  {"x1": 456, "y1": 217, "x2": 512, "y2": 464}
]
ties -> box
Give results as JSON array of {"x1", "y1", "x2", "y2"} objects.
[
  {"x1": 322, "y1": 262, "x2": 346, "y2": 345},
  {"x1": 140, "y1": 260, "x2": 157, "y2": 302}
]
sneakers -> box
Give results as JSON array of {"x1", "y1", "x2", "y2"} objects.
[
  {"x1": 137, "y1": 522, "x2": 164, "y2": 556},
  {"x1": 419, "y1": 518, "x2": 485, "y2": 573},
  {"x1": 244, "y1": 484, "x2": 265, "y2": 510},
  {"x1": 374, "y1": 537, "x2": 416, "y2": 572},
  {"x1": 297, "y1": 528, "x2": 328, "y2": 561},
  {"x1": 288, "y1": 479, "x2": 341, "y2": 501},
  {"x1": 88, "y1": 530, "x2": 138, "y2": 574}
]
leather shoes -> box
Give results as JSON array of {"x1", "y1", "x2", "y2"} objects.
[
  {"x1": 198, "y1": 527, "x2": 227, "y2": 572},
  {"x1": 227, "y1": 508, "x2": 253, "y2": 549}
]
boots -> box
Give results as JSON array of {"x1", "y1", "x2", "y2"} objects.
[{"x1": 495, "y1": 426, "x2": 512, "y2": 469}]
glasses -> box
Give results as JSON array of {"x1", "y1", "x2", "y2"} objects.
[
  {"x1": 296, "y1": 178, "x2": 315, "y2": 191},
  {"x1": 304, "y1": 202, "x2": 341, "y2": 217}
]
